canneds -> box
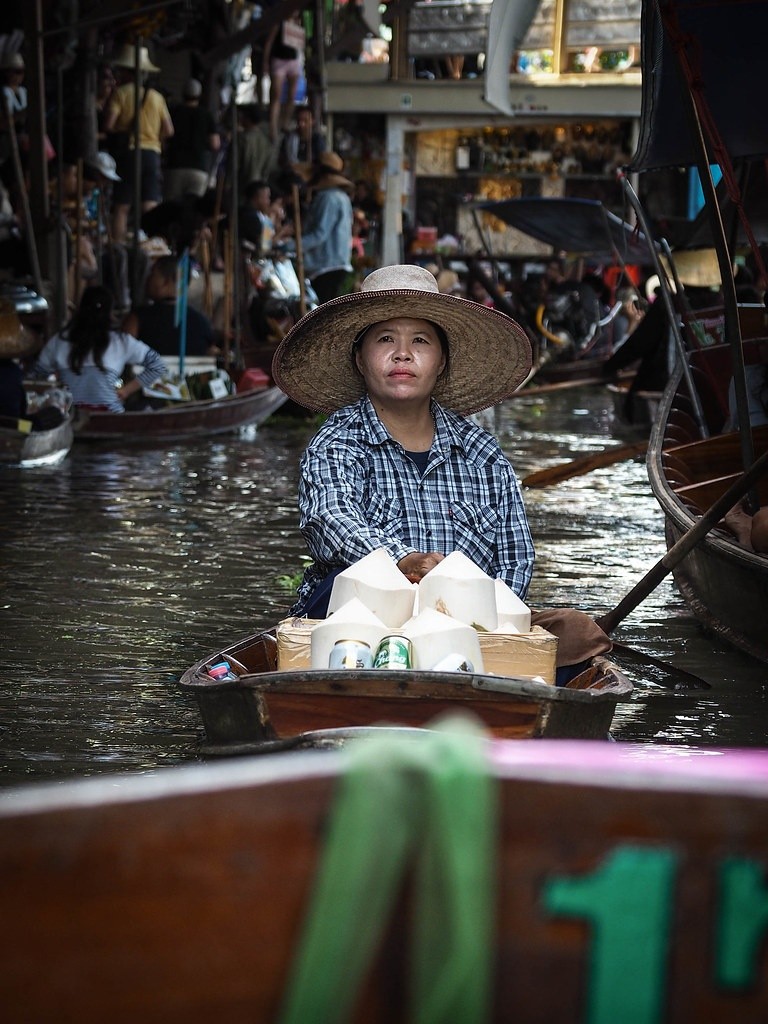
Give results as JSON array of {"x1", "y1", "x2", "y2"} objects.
[
  {"x1": 327, "y1": 638, "x2": 374, "y2": 669},
  {"x1": 372, "y1": 634, "x2": 412, "y2": 669}
]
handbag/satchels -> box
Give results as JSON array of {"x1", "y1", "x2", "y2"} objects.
[
  {"x1": 105, "y1": 130, "x2": 129, "y2": 168},
  {"x1": 271, "y1": 24, "x2": 297, "y2": 60}
]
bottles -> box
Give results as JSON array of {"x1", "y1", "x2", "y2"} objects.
[{"x1": 209, "y1": 662, "x2": 236, "y2": 681}]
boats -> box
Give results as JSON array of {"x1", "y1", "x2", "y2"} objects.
[
  {"x1": 606, "y1": 301, "x2": 767, "y2": 443},
  {"x1": 1, "y1": 364, "x2": 77, "y2": 468},
  {"x1": 75, "y1": 378, "x2": 295, "y2": 443},
  {"x1": 177, "y1": 582, "x2": 634, "y2": 770},
  {"x1": 220, "y1": 287, "x2": 727, "y2": 400},
  {"x1": 645, "y1": 336, "x2": 767, "y2": 667}
]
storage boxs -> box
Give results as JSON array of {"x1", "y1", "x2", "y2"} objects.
[{"x1": 277, "y1": 616, "x2": 559, "y2": 686}]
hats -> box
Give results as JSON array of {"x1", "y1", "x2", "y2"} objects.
[
  {"x1": 271, "y1": 265, "x2": 532, "y2": 416},
  {"x1": 291, "y1": 151, "x2": 355, "y2": 194},
  {"x1": 110, "y1": 43, "x2": 161, "y2": 73}
]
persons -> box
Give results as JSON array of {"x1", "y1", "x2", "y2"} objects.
[
  {"x1": 272, "y1": 262, "x2": 536, "y2": 622},
  {"x1": 1, "y1": 0, "x2": 767, "y2": 433}
]
